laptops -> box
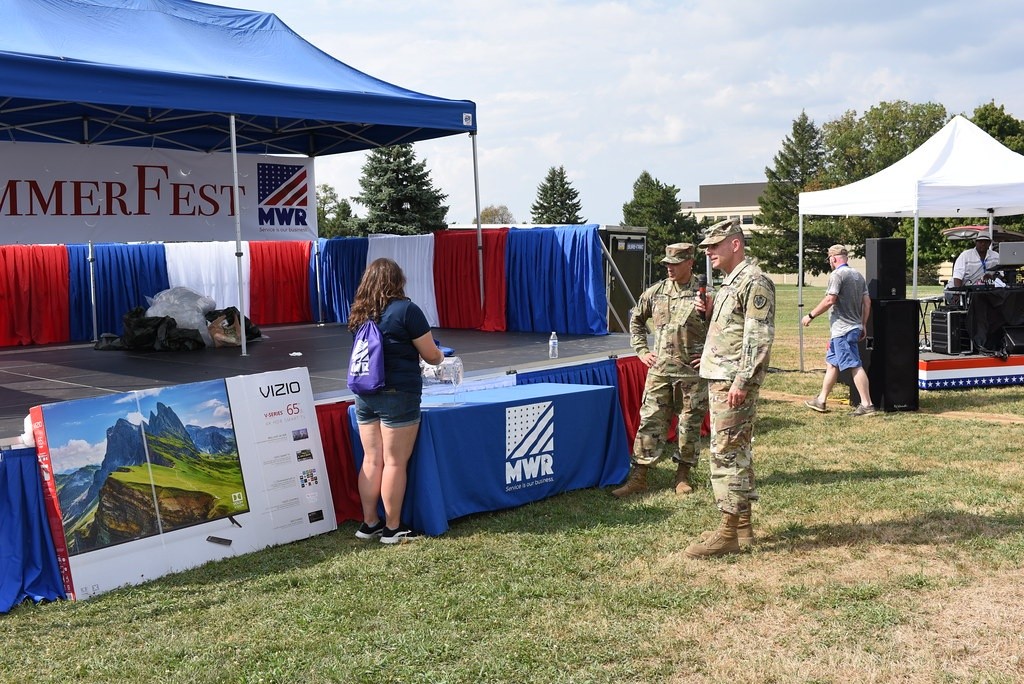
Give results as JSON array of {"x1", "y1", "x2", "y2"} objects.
[{"x1": 987, "y1": 242, "x2": 1024, "y2": 271}]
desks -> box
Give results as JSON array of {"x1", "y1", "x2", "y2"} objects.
[
  {"x1": 347, "y1": 382, "x2": 630, "y2": 536},
  {"x1": 944, "y1": 289, "x2": 1024, "y2": 353}
]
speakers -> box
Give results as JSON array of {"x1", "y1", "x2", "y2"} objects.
[
  {"x1": 999, "y1": 325, "x2": 1024, "y2": 354},
  {"x1": 838, "y1": 237, "x2": 920, "y2": 413}
]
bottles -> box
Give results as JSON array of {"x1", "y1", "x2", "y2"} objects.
[{"x1": 549, "y1": 332, "x2": 558, "y2": 358}]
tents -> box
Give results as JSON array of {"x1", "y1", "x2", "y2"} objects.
[
  {"x1": 799, "y1": 114, "x2": 1024, "y2": 374},
  {"x1": 0, "y1": 0, "x2": 484, "y2": 355}
]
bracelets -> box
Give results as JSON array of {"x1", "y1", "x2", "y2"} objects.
[{"x1": 809, "y1": 313, "x2": 814, "y2": 319}]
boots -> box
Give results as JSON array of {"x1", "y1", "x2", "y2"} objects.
[
  {"x1": 676, "y1": 463, "x2": 693, "y2": 494},
  {"x1": 611, "y1": 465, "x2": 649, "y2": 497},
  {"x1": 685, "y1": 513, "x2": 753, "y2": 559}
]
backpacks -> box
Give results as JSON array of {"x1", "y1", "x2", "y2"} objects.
[{"x1": 346, "y1": 321, "x2": 386, "y2": 396}]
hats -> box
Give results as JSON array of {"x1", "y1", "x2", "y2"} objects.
[
  {"x1": 825, "y1": 244, "x2": 848, "y2": 261},
  {"x1": 660, "y1": 242, "x2": 694, "y2": 265},
  {"x1": 698, "y1": 218, "x2": 742, "y2": 249},
  {"x1": 977, "y1": 231, "x2": 992, "y2": 241}
]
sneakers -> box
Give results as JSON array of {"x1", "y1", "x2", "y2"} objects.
[
  {"x1": 380, "y1": 524, "x2": 425, "y2": 543},
  {"x1": 805, "y1": 400, "x2": 827, "y2": 412},
  {"x1": 853, "y1": 403, "x2": 877, "y2": 417},
  {"x1": 354, "y1": 522, "x2": 383, "y2": 540}
]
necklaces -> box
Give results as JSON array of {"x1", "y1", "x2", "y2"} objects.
[{"x1": 837, "y1": 263, "x2": 849, "y2": 269}]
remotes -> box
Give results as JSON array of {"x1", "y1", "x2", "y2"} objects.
[{"x1": 206, "y1": 535, "x2": 232, "y2": 545}]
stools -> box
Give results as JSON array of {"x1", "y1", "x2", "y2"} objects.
[{"x1": 918, "y1": 298, "x2": 943, "y2": 346}]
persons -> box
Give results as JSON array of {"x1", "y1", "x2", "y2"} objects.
[
  {"x1": 944, "y1": 231, "x2": 1004, "y2": 305},
  {"x1": 801, "y1": 244, "x2": 876, "y2": 416},
  {"x1": 613, "y1": 243, "x2": 714, "y2": 497},
  {"x1": 683, "y1": 218, "x2": 776, "y2": 559},
  {"x1": 346, "y1": 257, "x2": 444, "y2": 543}
]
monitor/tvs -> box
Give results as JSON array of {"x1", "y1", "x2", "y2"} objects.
[{"x1": 42, "y1": 378, "x2": 251, "y2": 558}]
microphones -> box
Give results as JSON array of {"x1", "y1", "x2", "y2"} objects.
[{"x1": 698, "y1": 274, "x2": 706, "y2": 321}]
